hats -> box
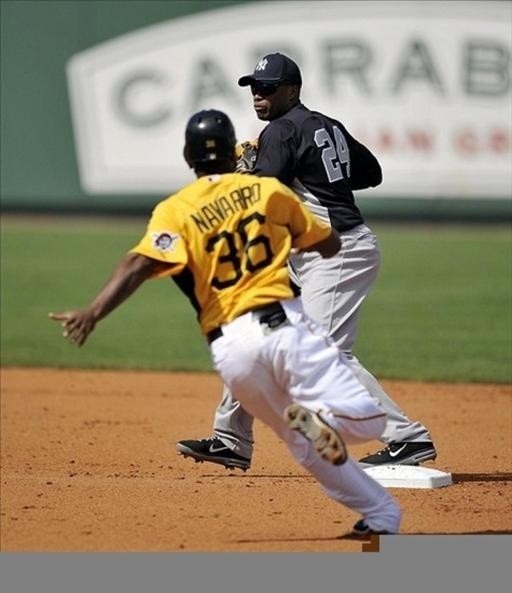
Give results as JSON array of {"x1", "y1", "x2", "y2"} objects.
[{"x1": 238, "y1": 53, "x2": 302, "y2": 86}]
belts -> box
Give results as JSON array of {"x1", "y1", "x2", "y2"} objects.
[{"x1": 206, "y1": 303, "x2": 281, "y2": 346}]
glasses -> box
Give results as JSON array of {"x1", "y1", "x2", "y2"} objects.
[{"x1": 251, "y1": 85, "x2": 277, "y2": 95}]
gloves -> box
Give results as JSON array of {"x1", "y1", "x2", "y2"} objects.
[{"x1": 236, "y1": 142, "x2": 261, "y2": 172}]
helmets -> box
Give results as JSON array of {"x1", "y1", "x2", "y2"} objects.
[{"x1": 183, "y1": 110, "x2": 235, "y2": 164}]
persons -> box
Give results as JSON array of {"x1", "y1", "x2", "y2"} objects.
[
  {"x1": 176, "y1": 50, "x2": 437, "y2": 473},
  {"x1": 48, "y1": 107, "x2": 403, "y2": 536}
]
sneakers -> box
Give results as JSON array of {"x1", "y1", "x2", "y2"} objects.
[
  {"x1": 353, "y1": 521, "x2": 396, "y2": 538},
  {"x1": 284, "y1": 406, "x2": 346, "y2": 465},
  {"x1": 176, "y1": 438, "x2": 250, "y2": 472},
  {"x1": 359, "y1": 441, "x2": 437, "y2": 466}
]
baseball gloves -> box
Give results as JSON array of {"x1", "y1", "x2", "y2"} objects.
[{"x1": 236, "y1": 139, "x2": 259, "y2": 173}]
